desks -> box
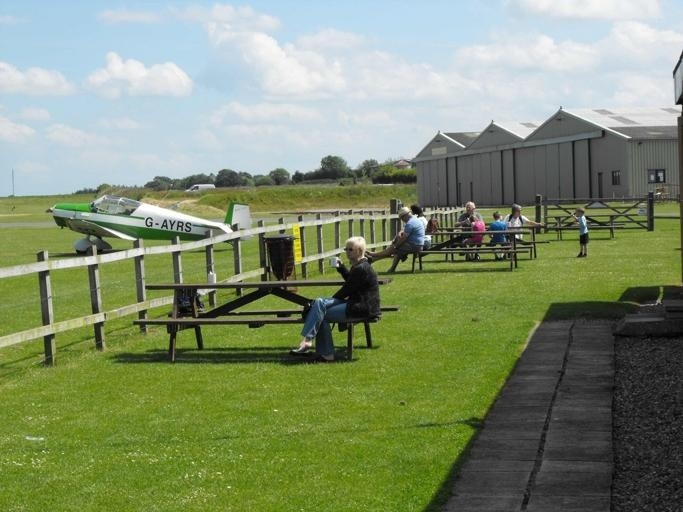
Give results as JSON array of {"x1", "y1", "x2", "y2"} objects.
[
  {"x1": 412, "y1": 225, "x2": 544, "y2": 273},
  {"x1": 145, "y1": 277, "x2": 394, "y2": 362}
]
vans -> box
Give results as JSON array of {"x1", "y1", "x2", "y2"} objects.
[{"x1": 184, "y1": 184, "x2": 215, "y2": 193}]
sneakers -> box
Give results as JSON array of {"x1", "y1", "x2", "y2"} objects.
[
  {"x1": 322, "y1": 354, "x2": 333, "y2": 361},
  {"x1": 291, "y1": 340, "x2": 314, "y2": 353},
  {"x1": 578, "y1": 252, "x2": 587, "y2": 257}
]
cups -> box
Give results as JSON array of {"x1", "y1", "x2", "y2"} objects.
[{"x1": 330, "y1": 256, "x2": 340, "y2": 269}]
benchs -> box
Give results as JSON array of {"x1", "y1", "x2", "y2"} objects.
[
  {"x1": 405, "y1": 241, "x2": 550, "y2": 254},
  {"x1": 168, "y1": 305, "x2": 402, "y2": 317},
  {"x1": 133, "y1": 314, "x2": 382, "y2": 324}
]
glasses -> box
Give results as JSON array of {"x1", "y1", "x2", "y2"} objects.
[{"x1": 344, "y1": 248, "x2": 354, "y2": 252}]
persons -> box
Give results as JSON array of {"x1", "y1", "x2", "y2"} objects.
[
  {"x1": 487, "y1": 212, "x2": 509, "y2": 261},
  {"x1": 454, "y1": 201, "x2": 486, "y2": 259},
  {"x1": 290, "y1": 235, "x2": 382, "y2": 362},
  {"x1": 364, "y1": 207, "x2": 425, "y2": 274},
  {"x1": 570, "y1": 207, "x2": 590, "y2": 258},
  {"x1": 405, "y1": 204, "x2": 432, "y2": 254},
  {"x1": 504, "y1": 203, "x2": 545, "y2": 243}
]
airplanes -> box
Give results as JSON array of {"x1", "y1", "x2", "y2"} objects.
[{"x1": 54, "y1": 194, "x2": 252, "y2": 256}]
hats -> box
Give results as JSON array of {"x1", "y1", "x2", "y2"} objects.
[{"x1": 398, "y1": 207, "x2": 409, "y2": 219}]
